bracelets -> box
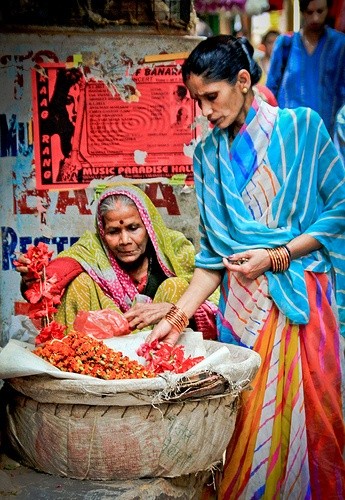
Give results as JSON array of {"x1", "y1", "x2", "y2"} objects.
[
  {"x1": 166, "y1": 301, "x2": 175, "y2": 306},
  {"x1": 266, "y1": 244, "x2": 293, "y2": 275},
  {"x1": 165, "y1": 306, "x2": 191, "y2": 334}
]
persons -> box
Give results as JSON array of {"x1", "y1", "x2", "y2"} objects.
[
  {"x1": 257, "y1": 29, "x2": 281, "y2": 89},
  {"x1": 265, "y1": 0, "x2": 345, "y2": 143},
  {"x1": 53, "y1": 182, "x2": 221, "y2": 339},
  {"x1": 143, "y1": 34, "x2": 345, "y2": 500}
]
